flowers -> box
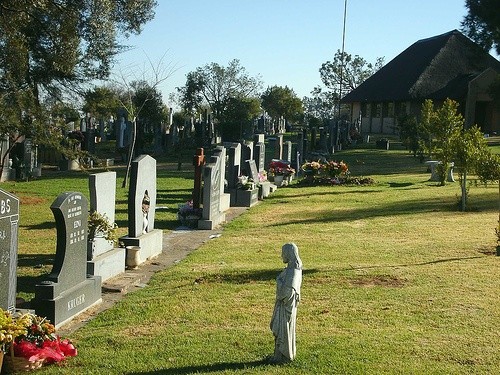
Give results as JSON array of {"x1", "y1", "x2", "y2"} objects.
[
  {"x1": 179, "y1": 200, "x2": 194, "y2": 225},
  {"x1": 0, "y1": 308, "x2": 78, "y2": 365},
  {"x1": 236, "y1": 159, "x2": 350, "y2": 190},
  {"x1": 87, "y1": 209, "x2": 120, "y2": 246}
]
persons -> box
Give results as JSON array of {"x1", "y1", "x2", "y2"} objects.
[{"x1": 269, "y1": 243, "x2": 304, "y2": 362}]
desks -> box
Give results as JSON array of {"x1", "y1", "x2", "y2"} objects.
[{"x1": 424, "y1": 160, "x2": 454, "y2": 181}]
